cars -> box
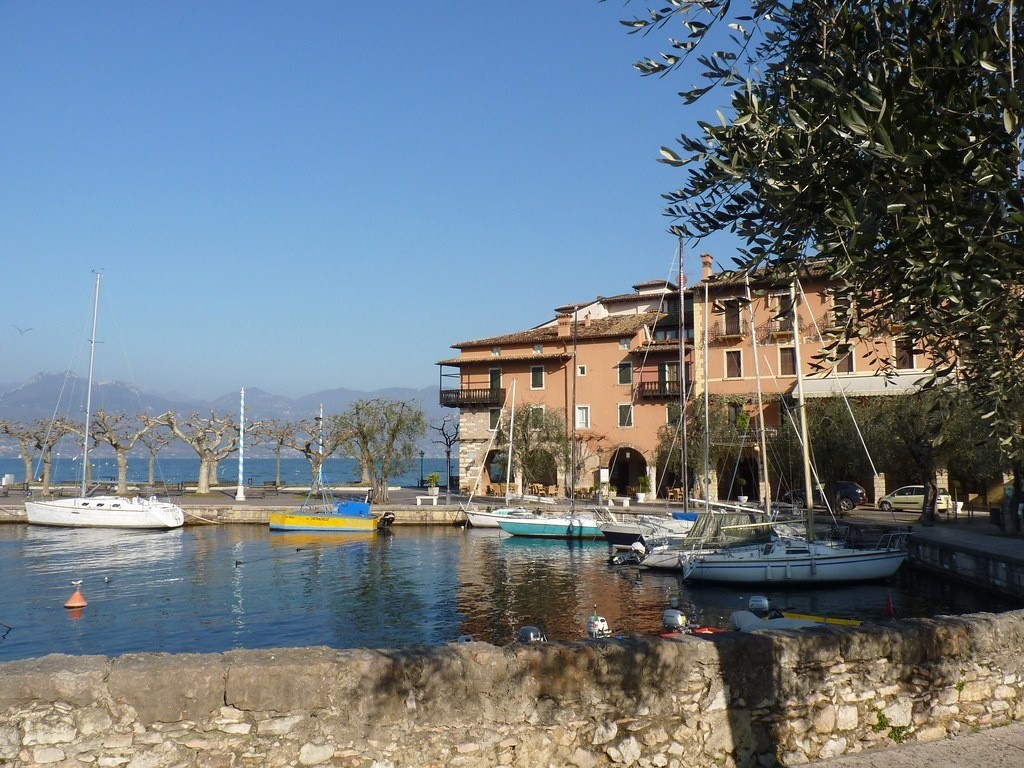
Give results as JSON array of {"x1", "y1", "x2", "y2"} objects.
[
  {"x1": 780, "y1": 479, "x2": 868, "y2": 511},
  {"x1": 877, "y1": 485, "x2": 953, "y2": 511}
]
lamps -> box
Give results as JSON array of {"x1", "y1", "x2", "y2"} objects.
[{"x1": 626, "y1": 450, "x2": 630, "y2": 461}]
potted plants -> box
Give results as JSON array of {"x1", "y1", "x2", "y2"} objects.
[
  {"x1": 427, "y1": 472, "x2": 440, "y2": 496},
  {"x1": 608, "y1": 476, "x2": 651, "y2": 503},
  {"x1": 737, "y1": 479, "x2": 749, "y2": 503},
  {"x1": 952, "y1": 480, "x2": 965, "y2": 512}
]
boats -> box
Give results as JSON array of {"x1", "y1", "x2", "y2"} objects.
[{"x1": 268, "y1": 507, "x2": 395, "y2": 533}]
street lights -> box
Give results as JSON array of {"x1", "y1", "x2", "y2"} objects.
[
  {"x1": 419, "y1": 449, "x2": 425, "y2": 487},
  {"x1": 315, "y1": 401, "x2": 329, "y2": 498},
  {"x1": 232, "y1": 386, "x2": 250, "y2": 502},
  {"x1": 445, "y1": 446, "x2": 453, "y2": 488}
]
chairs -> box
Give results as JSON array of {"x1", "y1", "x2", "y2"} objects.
[
  {"x1": 666, "y1": 486, "x2": 683, "y2": 502},
  {"x1": 460, "y1": 482, "x2": 593, "y2": 500}
]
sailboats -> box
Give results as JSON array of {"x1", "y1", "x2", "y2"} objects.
[
  {"x1": 497, "y1": 236, "x2": 691, "y2": 536},
  {"x1": 24, "y1": 267, "x2": 186, "y2": 532},
  {"x1": 616, "y1": 261, "x2": 908, "y2": 587},
  {"x1": 458, "y1": 375, "x2": 540, "y2": 528}
]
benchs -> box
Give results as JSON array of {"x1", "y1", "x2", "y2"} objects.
[
  {"x1": 733, "y1": 502, "x2": 759, "y2": 513},
  {"x1": 607, "y1": 496, "x2": 630, "y2": 507},
  {"x1": 2, "y1": 480, "x2": 287, "y2": 499}
]
